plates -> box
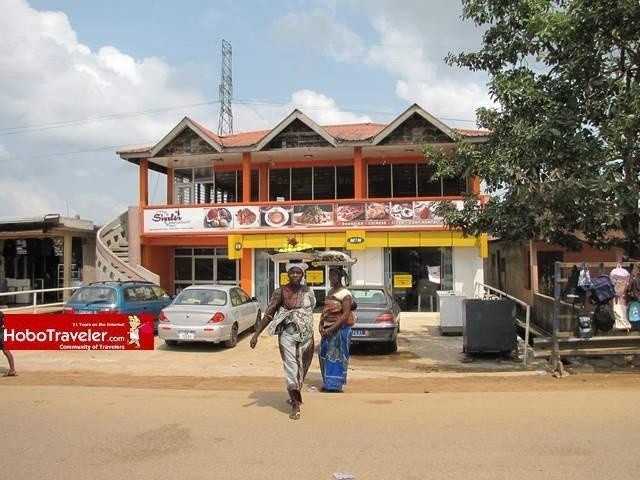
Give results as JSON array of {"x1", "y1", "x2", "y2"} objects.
[{"x1": 204, "y1": 204, "x2": 365, "y2": 229}]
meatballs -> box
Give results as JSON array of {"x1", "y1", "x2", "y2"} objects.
[{"x1": 212, "y1": 218, "x2": 227, "y2": 227}]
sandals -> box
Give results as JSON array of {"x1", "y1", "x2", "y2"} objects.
[{"x1": 287, "y1": 398, "x2": 300, "y2": 420}]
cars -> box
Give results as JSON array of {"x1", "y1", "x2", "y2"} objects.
[
  {"x1": 158, "y1": 284, "x2": 261, "y2": 348},
  {"x1": 62, "y1": 280, "x2": 174, "y2": 332},
  {"x1": 343, "y1": 285, "x2": 400, "y2": 352}
]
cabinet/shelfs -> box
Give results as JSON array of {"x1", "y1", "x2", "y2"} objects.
[{"x1": 57, "y1": 263, "x2": 80, "y2": 301}]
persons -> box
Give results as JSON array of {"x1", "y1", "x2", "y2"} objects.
[
  {"x1": 319, "y1": 267, "x2": 354, "y2": 393},
  {"x1": 249, "y1": 262, "x2": 317, "y2": 420},
  {"x1": 0, "y1": 311, "x2": 16, "y2": 377}
]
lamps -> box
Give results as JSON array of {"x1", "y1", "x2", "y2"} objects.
[
  {"x1": 211, "y1": 159, "x2": 217, "y2": 163},
  {"x1": 304, "y1": 155, "x2": 312, "y2": 158},
  {"x1": 404, "y1": 149, "x2": 414, "y2": 152}
]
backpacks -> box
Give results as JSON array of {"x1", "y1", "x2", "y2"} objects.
[
  {"x1": 562, "y1": 265, "x2": 586, "y2": 304},
  {"x1": 588, "y1": 263, "x2": 640, "y2": 336},
  {"x1": 574, "y1": 305, "x2": 596, "y2": 338}
]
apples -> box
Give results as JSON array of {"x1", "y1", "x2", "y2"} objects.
[{"x1": 279, "y1": 242, "x2": 312, "y2": 252}]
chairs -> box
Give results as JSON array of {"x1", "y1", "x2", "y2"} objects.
[
  {"x1": 416, "y1": 279, "x2": 439, "y2": 312},
  {"x1": 0, "y1": 279, "x2": 17, "y2": 305}
]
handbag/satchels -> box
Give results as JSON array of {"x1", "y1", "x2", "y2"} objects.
[{"x1": 577, "y1": 269, "x2": 593, "y2": 291}]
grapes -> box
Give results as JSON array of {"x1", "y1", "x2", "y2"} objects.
[{"x1": 287, "y1": 238, "x2": 298, "y2": 245}]
